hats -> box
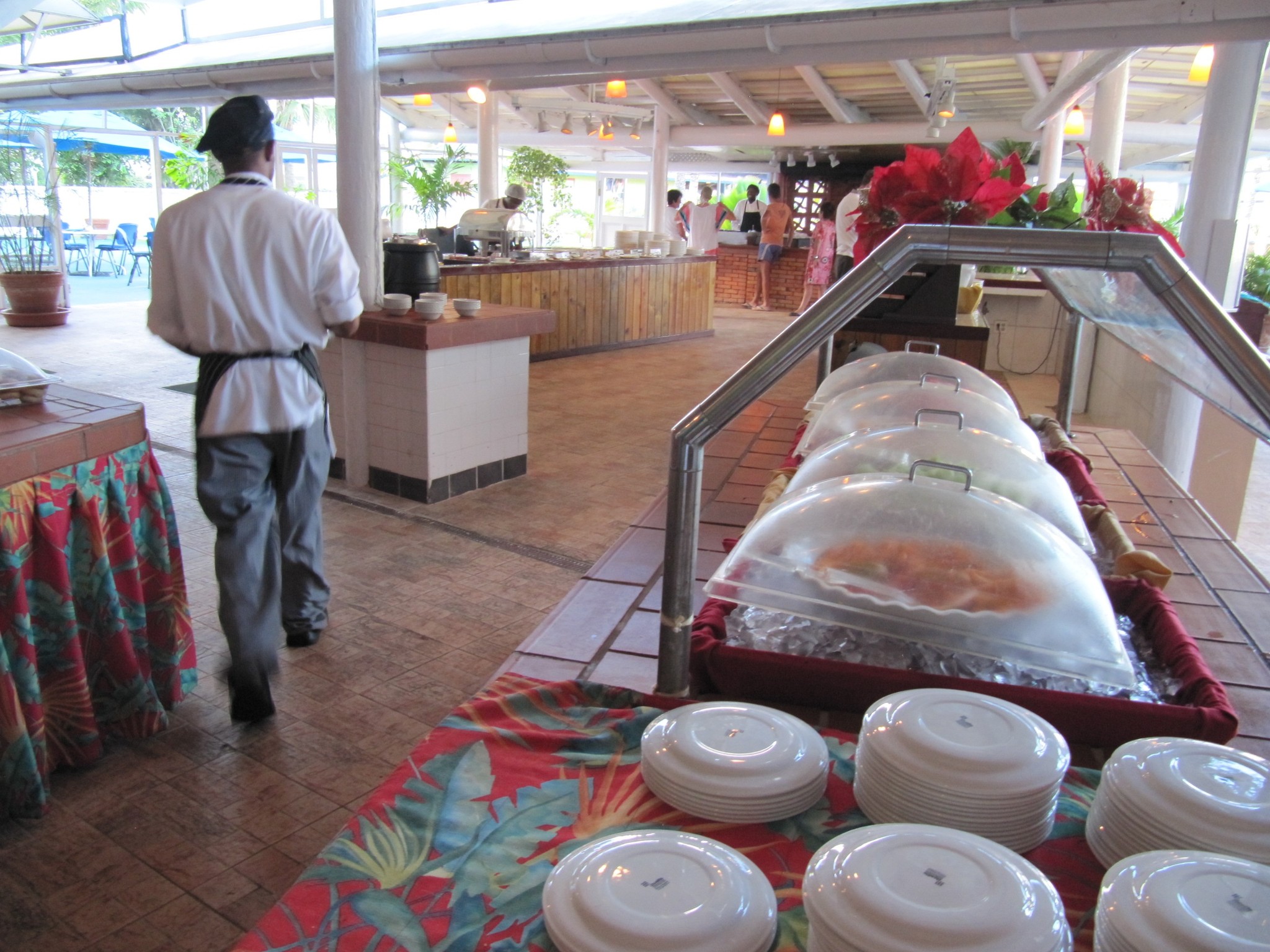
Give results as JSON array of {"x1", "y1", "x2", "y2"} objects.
[{"x1": 505, "y1": 184, "x2": 525, "y2": 201}]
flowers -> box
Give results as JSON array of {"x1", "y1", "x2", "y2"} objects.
[
  {"x1": 1061, "y1": 139, "x2": 1188, "y2": 260},
  {"x1": 843, "y1": 124, "x2": 1087, "y2": 274}
]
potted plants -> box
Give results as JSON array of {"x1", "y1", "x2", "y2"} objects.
[{"x1": 0, "y1": 110, "x2": 93, "y2": 326}]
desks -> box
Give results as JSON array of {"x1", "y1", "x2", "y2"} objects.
[
  {"x1": 233, "y1": 422, "x2": 1270, "y2": 952},
  {"x1": 0, "y1": 379, "x2": 197, "y2": 823},
  {"x1": 816, "y1": 312, "x2": 992, "y2": 376}
]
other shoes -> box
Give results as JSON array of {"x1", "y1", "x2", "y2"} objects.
[
  {"x1": 286, "y1": 629, "x2": 321, "y2": 647},
  {"x1": 788, "y1": 312, "x2": 801, "y2": 317},
  {"x1": 229, "y1": 672, "x2": 276, "y2": 721}
]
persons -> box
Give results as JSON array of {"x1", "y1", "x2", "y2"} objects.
[
  {"x1": 667, "y1": 190, "x2": 687, "y2": 243},
  {"x1": 731, "y1": 184, "x2": 768, "y2": 231},
  {"x1": 742, "y1": 183, "x2": 795, "y2": 312},
  {"x1": 833, "y1": 165, "x2": 875, "y2": 284},
  {"x1": 146, "y1": 95, "x2": 363, "y2": 720},
  {"x1": 788, "y1": 203, "x2": 837, "y2": 316},
  {"x1": 676, "y1": 187, "x2": 737, "y2": 255},
  {"x1": 479, "y1": 183, "x2": 525, "y2": 253}
]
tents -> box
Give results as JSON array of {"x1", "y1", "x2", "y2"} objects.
[{"x1": 0, "y1": 109, "x2": 207, "y2": 310}]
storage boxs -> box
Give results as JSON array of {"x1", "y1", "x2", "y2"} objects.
[
  {"x1": 687, "y1": 557, "x2": 1240, "y2": 766},
  {"x1": 747, "y1": 233, "x2": 762, "y2": 245},
  {"x1": 955, "y1": 285, "x2": 984, "y2": 314},
  {"x1": 782, "y1": 236, "x2": 811, "y2": 249},
  {"x1": 722, "y1": 414, "x2": 1149, "y2": 589}
]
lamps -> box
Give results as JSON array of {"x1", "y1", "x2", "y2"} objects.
[
  {"x1": 926, "y1": 122, "x2": 940, "y2": 138},
  {"x1": 767, "y1": 67, "x2": 785, "y2": 136},
  {"x1": 936, "y1": 90, "x2": 957, "y2": 118},
  {"x1": 413, "y1": 94, "x2": 433, "y2": 107},
  {"x1": 444, "y1": 93, "x2": 458, "y2": 142},
  {"x1": 598, "y1": 114, "x2": 615, "y2": 140},
  {"x1": 807, "y1": 153, "x2": 816, "y2": 167},
  {"x1": 560, "y1": 110, "x2": 574, "y2": 134},
  {"x1": 786, "y1": 152, "x2": 796, "y2": 167},
  {"x1": 1186, "y1": 44, "x2": 1216, "y2": 84},
  {"x1": 583, "y1": 113, "x2": 599, "y2": 136},
  {"x1": 629, "y1": 118, "x2": 643, "y2": 140},
  {"x1": 604, "y1": 80, "x2": 627, "y2": 99},
  {"x1": 537, "y1": 110, "x2": 551, "y2": 133},
  {"x1": 1062, "y1": 49, "x2": 1088, "y2": 135},
  {"x1": 931, "y1": 109, "x2": 946, "y2": 128},
  {"x1": 601, "y1": 117, "x2": 614, "y2": 136},
  {"x1": 467, "y1": 84, "x2": 488, "y2": 104},
  {"x1": 828, "y1": 152, "x2": 841, "y2": 168},
  {"x1": 767, "y1": 151, "x2": 778, "y2": 168}
]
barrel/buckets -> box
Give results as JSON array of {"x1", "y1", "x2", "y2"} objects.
[{"x1": 381, "y1": 241, "x2": 440, "y2": 301}]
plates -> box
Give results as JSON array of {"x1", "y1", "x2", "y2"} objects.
[
  {"x1": 511, "y1": 255, "x2": 662, "y2": 263},
  {"x1": 614, "y1": 230, "x2": 705, "y2": 258},
  {"x1": 542, "y1": 684, "x2": 1268, "y2": 952},
  {"x1": 490, "y1": 261, "x2": 515, "y2": 264}
]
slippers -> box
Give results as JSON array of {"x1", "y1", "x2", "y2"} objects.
[
  {"x1": 752, "y1": 306, "x2": 772, "y2": 311},
  {"x1": 742, "y1": 302, "x2": 753, "y2": 309}
]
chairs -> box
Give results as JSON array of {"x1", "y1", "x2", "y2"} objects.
[
  {"x1": 35, "y1": 221, "x2": 91, "y2": 274},
  {"x1": 95, "y1": 222, "x2": 142, "y2": 279},
  {"x1": 126, "y1": 230, "x2": 157, "y2": 290}
]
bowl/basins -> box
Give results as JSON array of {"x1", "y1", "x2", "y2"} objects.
[
  {"x1": 383, "y1": 292, "x2": 480, "y2": 321},
  {"x1": 495, "y1": 257, "x2": 511, "y2": 262}
]
